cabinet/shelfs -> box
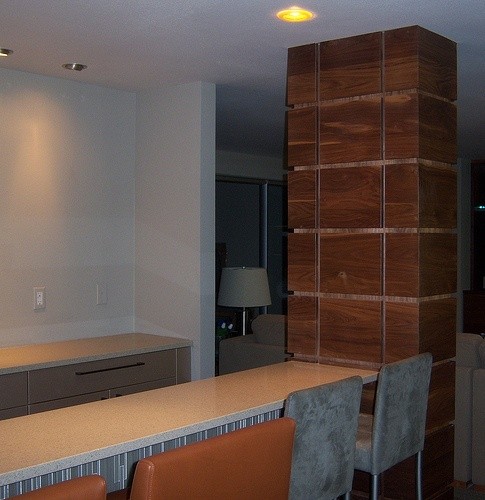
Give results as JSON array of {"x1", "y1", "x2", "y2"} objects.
[{"x1": 0, "y1": 334, "x2": 194, "y2": 418}]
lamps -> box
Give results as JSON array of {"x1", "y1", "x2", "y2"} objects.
[
  {"x1": 63, "y1": 64, "x2": 84, "y2": 72},
  {"x1": 0, "y1": 48, "x2": 9, "y2": 56},
  {"x1": 217, "y1": 266, "x2": 272, "y2": 336}
]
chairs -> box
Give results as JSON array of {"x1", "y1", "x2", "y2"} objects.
[
  {"x1": 107, "y1": 416, "x2": 297, "y2": 499},
  {"x1": 5, "y1": 475, "x2": 108, "y2": 500},
  {"x1": 354, "y1": 354, "x2": 434, "y2": 500},
  {"x1": 286, "y1": 375, "x2": 362, "y2": 500}
]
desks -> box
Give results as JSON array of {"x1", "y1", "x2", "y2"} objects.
[{"x1": 0, "y1": 360, "x2": 387, "y2": 500}]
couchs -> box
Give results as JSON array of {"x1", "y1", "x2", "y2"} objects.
[
  {"x1": 455, "y1": 333, "x2": 485, "y2": 487},
  {"x1": 218, "y1": 313, "x2": 287, "y2": 373}
]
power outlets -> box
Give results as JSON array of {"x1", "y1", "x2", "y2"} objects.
[{"x1": 32, "y1": 287, "x2": 46, "y2": 310}]
